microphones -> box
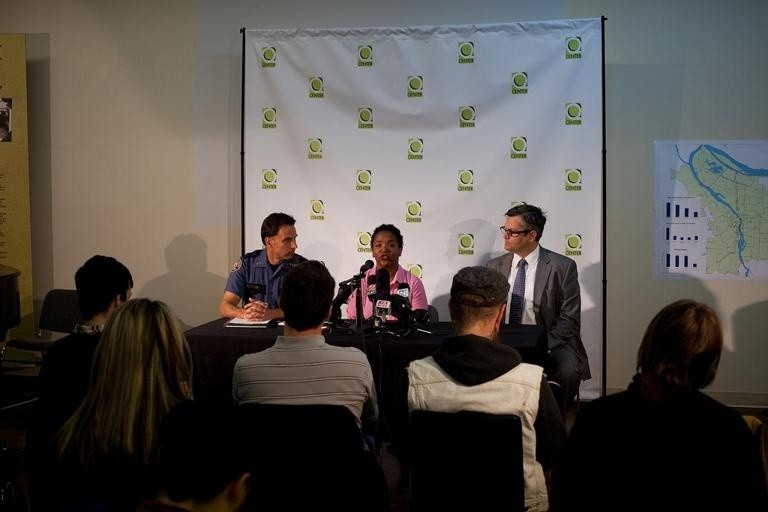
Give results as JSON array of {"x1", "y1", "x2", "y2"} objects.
[{"x1": 333, "y1": 260, "x2": 413, "y2": 333}]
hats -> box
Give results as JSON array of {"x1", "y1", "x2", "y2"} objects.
[{"x1": 451, "y1": 266, "x2": 511, "y2": 308}]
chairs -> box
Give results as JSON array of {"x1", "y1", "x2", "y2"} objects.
[
  {"x1": 2, "y1": 288, "x2": 80, "y2": 367},
  {"x1": 423, "y1": 305, "x2": 439, "y2": 322}
]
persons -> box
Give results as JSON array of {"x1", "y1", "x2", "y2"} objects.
[
  {"x1": 130, "y1": 398, "x2": 258, "y2": 511},
  {"x1": 25, "y1": 254, "x2": 133, "y2": 512},
  {"x1": 566, "y1": 298, "x2": 758, "y2": 435},
  {"x1": 34, "y1": 296, "x2": 199, "y2": 511},
  {"x1": 485, "y1": 204, "x2": 591, "y2": 417},
  {"x1": 219, "y1": 213, "x2": 308, "y2": 321},
  {"x1": 230, "y1": 260, "x2": 380, "y2": 456},
  {"x1": 339, "y1": 223, "x2": 428, "y2": 322},
  {"x1": 392, "y1": 265, "x2": 564, "y2": 511}
]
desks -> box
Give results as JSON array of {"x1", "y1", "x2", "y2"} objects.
[
  {"x1": 0, "y1": 264, "x2": 22, "y2": 341},
  {"x1": 181, "y1": 312, "x2": 546, "y2": 396}
]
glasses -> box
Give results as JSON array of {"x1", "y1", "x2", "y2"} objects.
[{"x1": 500, "y1": 226, "x2": 531, "y2": 237}]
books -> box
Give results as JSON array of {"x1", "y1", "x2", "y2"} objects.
[{"x1": 223, "y1": 318, "x2": 274, "y2": 329}]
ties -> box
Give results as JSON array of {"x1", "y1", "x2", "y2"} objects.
[{"x1": 509, "y1": 258, "x2": 528, "y2": 326}]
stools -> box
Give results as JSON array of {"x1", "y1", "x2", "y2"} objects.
[{"x1": 547, "y1": 373, "x2": 581, "y2": 426}]
